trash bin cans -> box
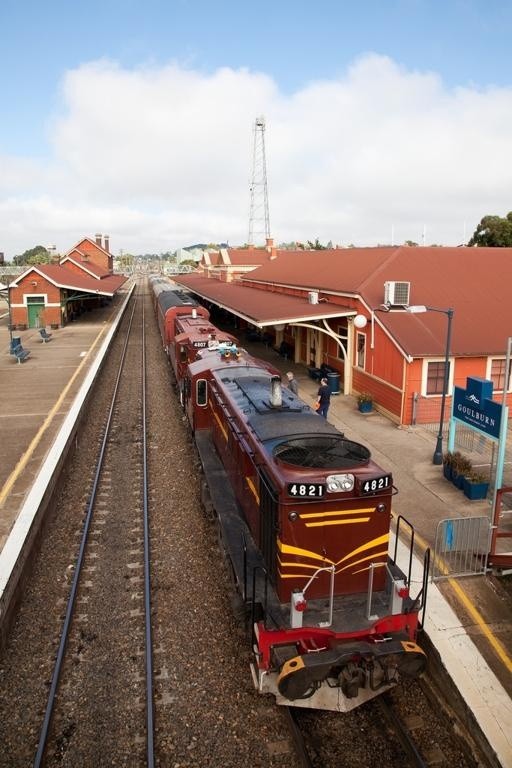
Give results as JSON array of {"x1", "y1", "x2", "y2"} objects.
[
  {"x1": 327, "y1": 373, "x2": 340, "y2": 395},
  {"x1": 13, "y1": 337, "x2": 21, "y2": 353}
]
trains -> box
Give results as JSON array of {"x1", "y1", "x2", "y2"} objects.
[{"x1": 148, "y1": 273, "x2": 429, "y2": 713}]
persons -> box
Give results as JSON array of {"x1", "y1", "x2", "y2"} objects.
[
  {"x1": 315, "y1": 377, "x2": 330, "y2": 418},
  {"x1": 286, "y1": 371, "x2": 298, "y2": 395}
]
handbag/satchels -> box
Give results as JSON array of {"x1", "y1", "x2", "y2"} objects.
[{"x1": 313, "y1": 401, "x2": 321, "y2": 410}]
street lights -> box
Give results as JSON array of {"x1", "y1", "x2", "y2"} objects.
[
  {"x1": 406, "y1": 304, "x2": 453, "y2": 465},
  {"x1": 7, "y1": 279, "x2": 18, "y2": 340}
]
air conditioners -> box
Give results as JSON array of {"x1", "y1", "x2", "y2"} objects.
[
  {"x1": 385, "y1": 280, "x2": 411, "y2": 306},
  {"x1": 309, "y1": 291, "x2": 318, "y2": 305}
]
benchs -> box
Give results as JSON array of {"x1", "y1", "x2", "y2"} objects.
[
  {"x1": 260, "y1": 331, "x2": 274, "y2": 348},
  {"x1": 278, "y1": 340, "x2": 295, "y2": 359},
  {"x1": 13, "y1": 342, "x2": 30, "y2": 363},
  {"x1": 37, "y1": 328, "x2": 52, "y2": 343},
  {"x1": 308, "y1": 362, "x2": 340, "y2": 383}
]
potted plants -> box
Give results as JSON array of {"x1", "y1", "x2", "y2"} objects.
[
  {"x1": 442, "y1": 450, "x2": 490, "y2": 500},
  {"x1": 355, "y1": 390, "x2": 373, "y2": 413}
]
